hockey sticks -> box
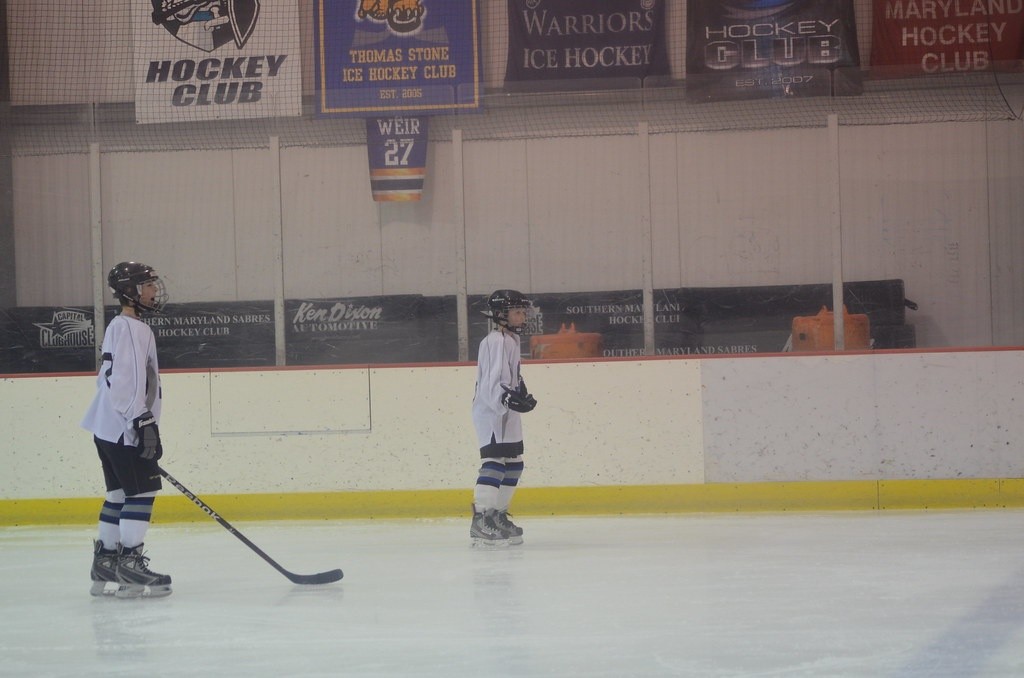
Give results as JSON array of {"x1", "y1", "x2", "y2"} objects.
[
  {"x1": 158, "y1": 467, "x2": 344, "y2": 585},
  {"x1": 501, "y1": 384, "x2": 533, "y2": 407}
]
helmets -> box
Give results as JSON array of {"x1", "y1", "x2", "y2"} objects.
[
  {"x1": 488, "y1": 290, "x2": 531, "y2": 336},
  {"x1": 107, "y1": 261, "x2": 169, "y2": 318}
]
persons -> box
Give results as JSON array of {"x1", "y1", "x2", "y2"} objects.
[
  {"x1": 80, "y1": 263, "x2": 174, "y2": 590},
  {"x1": 469, "y1": 290, "x2": 538, "y2": 541}
]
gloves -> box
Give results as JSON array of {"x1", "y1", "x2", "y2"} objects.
[
  {"x1": 133, "y1": 411, "x2": 163, "y2": 461},
  {"x1": 501, "y1": 391, "x2": 537, "y2": 413}
]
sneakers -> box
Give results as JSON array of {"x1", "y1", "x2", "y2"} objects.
[
  {"x1": 90, "y1": 539, "x2": 119, "y2": 596},
  {"x1": 492, "y1": 509, "x2": 524, "y2": 545},
  {"x1": 470, "y1": 503, "x2": 512, "y2": 551},
  {"x1": 114, "y1": 542, "x2": 172, "y2": 599}
]
